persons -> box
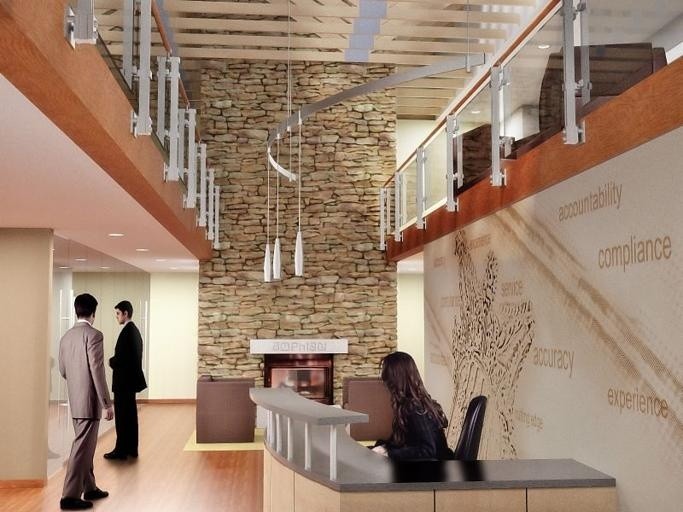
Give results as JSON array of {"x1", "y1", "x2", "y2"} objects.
[
  {"x1": 370, "y1": 350, "x2": 449, "y2": 460},
  {"x1": 58, "y1": 293, "x2": 113, "y2": 509},
  {"x1": 102, "y1": 300, "x2": 147, "y2": 460}
]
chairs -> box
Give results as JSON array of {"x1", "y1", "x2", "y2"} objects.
[
  {"x1": 452, "y1": 395, "x2": 487, "y2": 460},
  {"x1": 197, "y1": 374, "x2": 255, "y2": 443},
  {"x1": 342, "y1": 377, "x2": 394, "y2": 441}
]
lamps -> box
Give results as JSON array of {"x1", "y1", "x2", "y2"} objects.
[{"x1": 264, "y1": 118, "x2": 303, "y2": 283}]
